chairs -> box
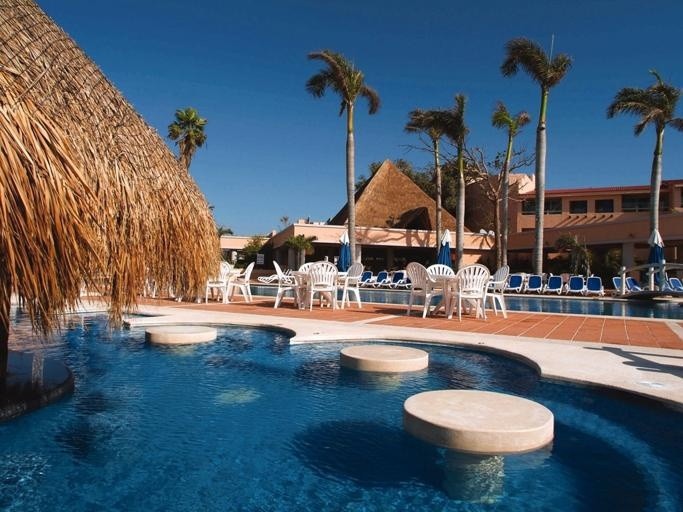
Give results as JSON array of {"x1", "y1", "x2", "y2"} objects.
[
  {"x1": 503, "y1": 274, "x2": 682, "y2": 299},
  {"x1": 360, "y1": 270, "x2": 412, "y2": 291},
  {"x1": 272, "y1": 259, "x2": 362, "y2": 313},
  {"x1": 171, "y1": 260, "x2": 255, "y2": 305},
  {"x1": 405, "y1": 261, "x2": 509, "y2": 322}
]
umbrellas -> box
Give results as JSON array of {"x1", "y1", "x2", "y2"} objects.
[
  {"x1": 643, "y1": 227, "x2": 667, "y2": 284},
  {"x1": 435, "y1": 228, "x2": 453, "y2": 269},
  {"x1": 337, "y1": 230, "x2": 352, "y2": 271}
]
